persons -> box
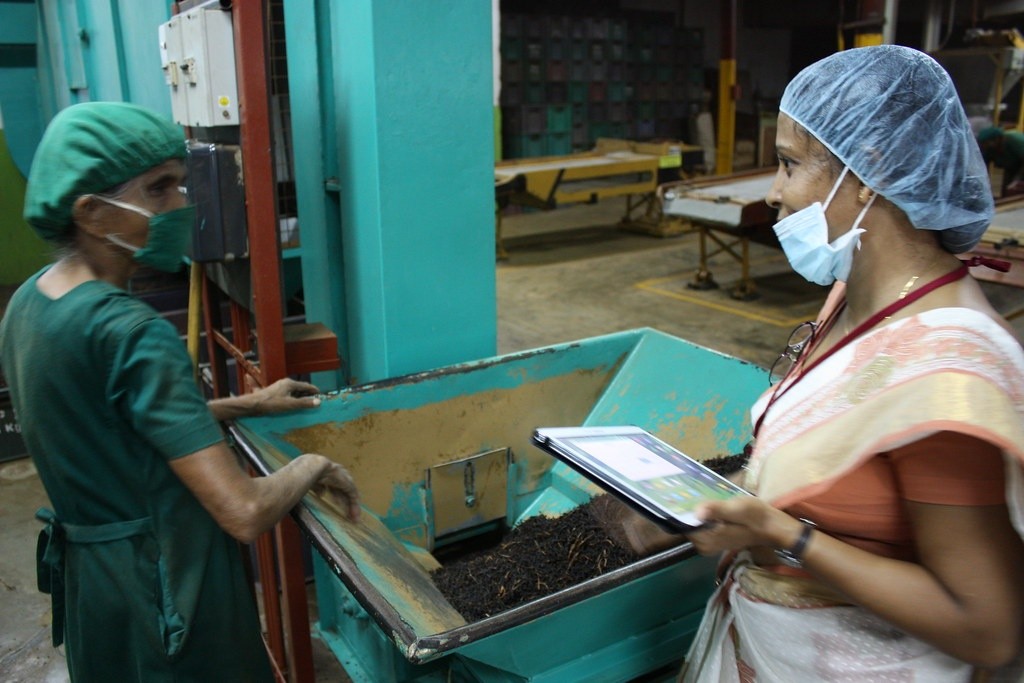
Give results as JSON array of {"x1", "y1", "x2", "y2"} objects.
[
  {"x1": 1, "y1": 98, "x2": 364, "y2": 683},
  {"x1": 680, "y1": 44, "x2": 1024, "y2": 683}
]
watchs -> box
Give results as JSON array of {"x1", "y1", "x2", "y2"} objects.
[{"x1": 774, "y1": 516, "x2": 817, "y2": 571}]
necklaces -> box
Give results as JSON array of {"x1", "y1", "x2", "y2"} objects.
[
  {"x1": 739, "y1": 255, "x2": 972, "y2": 464},
  {"x1": 840, "y1": 241, "x2": 944, "y2": 342}
]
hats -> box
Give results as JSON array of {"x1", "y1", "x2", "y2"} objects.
[{"x1": 24, "y1": 101, "x2": 188, "y2": 240}]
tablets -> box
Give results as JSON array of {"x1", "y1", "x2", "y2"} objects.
[{"x1": 531, "y1": 425, "x2": 758, "y2": 529}]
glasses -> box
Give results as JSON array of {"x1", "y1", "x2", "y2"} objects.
[{"x1": 768, "y1": 320, "x2": 818, "y2": 387}]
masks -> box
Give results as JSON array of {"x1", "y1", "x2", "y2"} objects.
[
  {"x1": 772, "y1": 149, "x2": 893, "y2": 286},
  {"x1": 83, "y1": 194, "x2": 196, "y2": 273}
]
